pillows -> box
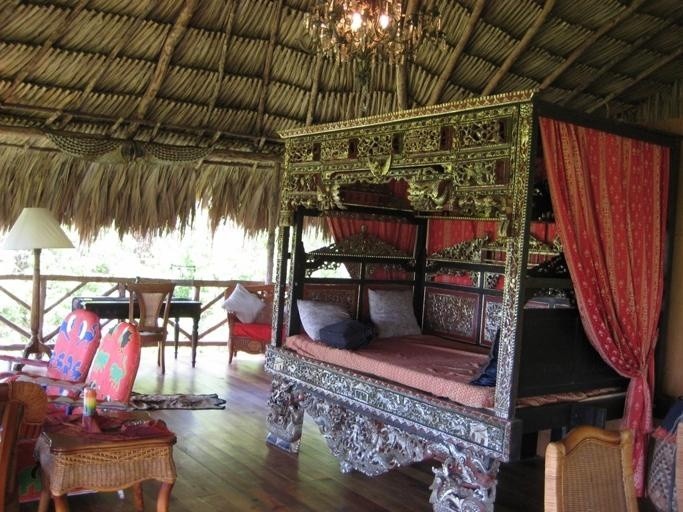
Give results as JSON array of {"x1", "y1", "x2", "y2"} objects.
[
  {"x1": 467, "y1": 326, "x2": 499, "y2": 386},
  {"x1": 221, "y1": 283, "x2": 264, "y2": 324},
  {"x1": 295, "y1": 298, "x2": 354, "y2": 340},
  {"x1": 368, "y1": 289, "x2": 422, "y2": 337}
]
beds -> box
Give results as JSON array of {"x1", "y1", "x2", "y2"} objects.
[{"x1": 263, "y1": 89, "x2": 683, "y2": 512}]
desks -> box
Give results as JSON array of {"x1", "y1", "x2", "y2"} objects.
[{"x1": 73, "y1": 299, "x2": 198, "y2": 368}]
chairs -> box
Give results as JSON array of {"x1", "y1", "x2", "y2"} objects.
[
  {"x1": 543, "y1": 423, "x2": 639, "y2": 512},
  {"x1": 226, "y1": 281, "x2": 275, "y2": 365},
  {"x1": 0, "y1": 309, "x2": 101, "y2": 433},
  {"x1": 0, "y1": 321, "x2": 140, "y2": 511},
  {"x1": 124, "y1": 284, "x2": 174, "y2": 375}
]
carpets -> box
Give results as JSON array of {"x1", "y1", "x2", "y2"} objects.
[{"x1": 127, "y1": 392, "x2": 226, "y2": 411}]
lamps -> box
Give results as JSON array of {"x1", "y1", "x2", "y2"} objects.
[
  {"x1": 0, "y1": 208, "x2": 76, "y2": 373},
  {"x1": 297, "y1": 0, "x2": 437, "y2": 63}
]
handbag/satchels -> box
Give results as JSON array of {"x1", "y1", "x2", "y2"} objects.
[{"x1": 318, "y1": 319, "x2": 374, "y2": 349}]
